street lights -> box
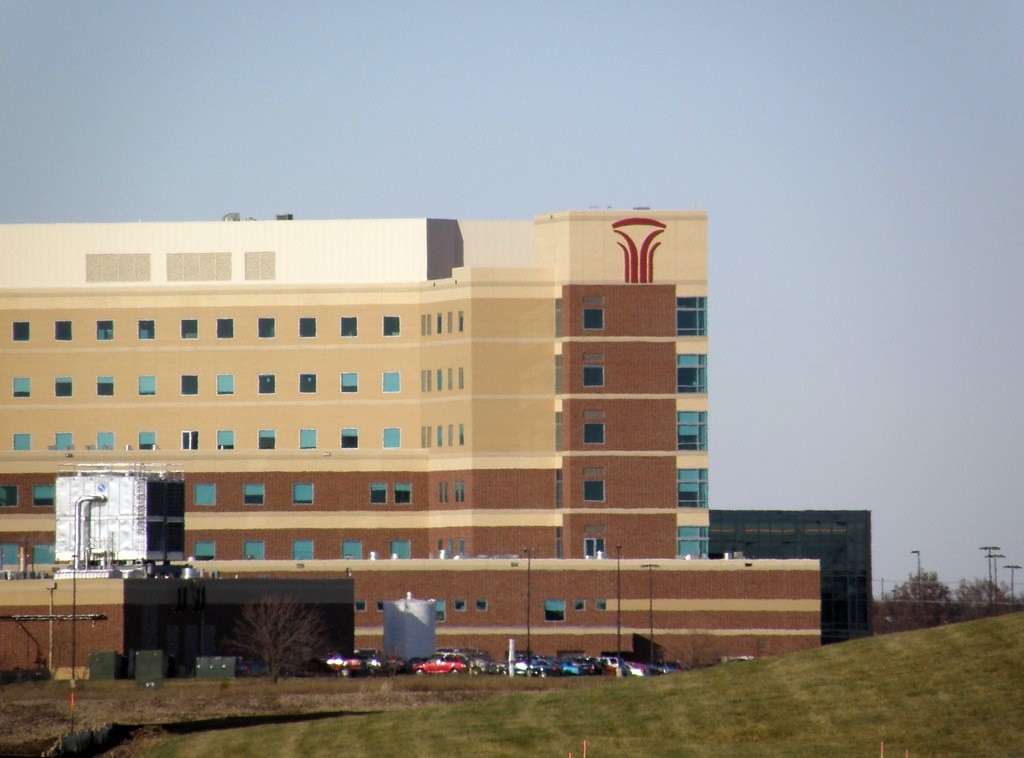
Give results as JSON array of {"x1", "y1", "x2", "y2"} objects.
[
  {"x1": 1003, "y1": 565, "x2": 1023, "y2": 613},
  {"x1": 979, "y1": 547, "x2": 1002, "y2": 617},
  {"x1": 520, "y1": 548, "x2": 532, "y2": 660},
  {"x1": 911, "y1": 550, "x2": 923, "y2": 623},
  {"x1": 640, "y1": 563, "x2": 660, "y2": 661},
  {"x1": 616, "y1": 544, "x2": 622, "y2": 659},
  {"x1": 988, "y1": 554, "x2": 1005, "y2": 612}
]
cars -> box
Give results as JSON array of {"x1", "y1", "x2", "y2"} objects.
[
  {"x1": 435, "y1": 647, "x2": 684, "y2": 679},
  {"x1": 322, "y1": 648, "x2": 406, "y2": 676},
  {"x1": 411, "y1": 654, "x2": 480, "y2": 675}
]
